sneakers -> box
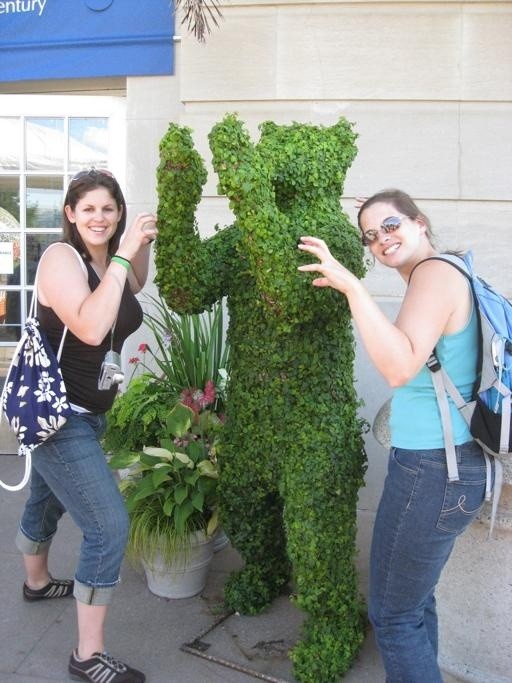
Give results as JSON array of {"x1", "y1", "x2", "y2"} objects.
[
  {"x1": 23, "y1": 571, "x2": 74, "y2": 602},
  {"x1": 68, "y1": 648, "x2": 146, "y2": 683}
]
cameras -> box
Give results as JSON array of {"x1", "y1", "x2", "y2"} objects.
[{"x1": 97, "y1": 349, "x2": 124, "y2": 391}]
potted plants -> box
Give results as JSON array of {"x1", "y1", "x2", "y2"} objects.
[{"x1": 98, "y1": 285, "x2": 231, "y2": 601}]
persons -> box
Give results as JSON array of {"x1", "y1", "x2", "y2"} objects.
[
  {"x1": 294, "y1": 188, "x2": 500, "y2": 683},
  {"x1": 15, "y1": 169, "x2": 160, "y2": 683}
]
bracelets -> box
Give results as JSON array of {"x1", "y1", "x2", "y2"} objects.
[{"x1": 111, "y1": 255, "x2": 131, "y2": 272}]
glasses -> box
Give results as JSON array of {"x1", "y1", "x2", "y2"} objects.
[
  {"x1": 71, "y1": 169, "x2": 116, "y2": 181},
  {"x1": 361, "y1": 214, "x2": 418, "y2": 247}
]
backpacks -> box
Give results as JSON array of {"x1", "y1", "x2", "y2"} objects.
[
  {"x1": 0, "y1": 241, "x2": 89, "y2": 492},
  {"x1": 406, "y1": 253, "x2": 512, "y2": 457}
]
